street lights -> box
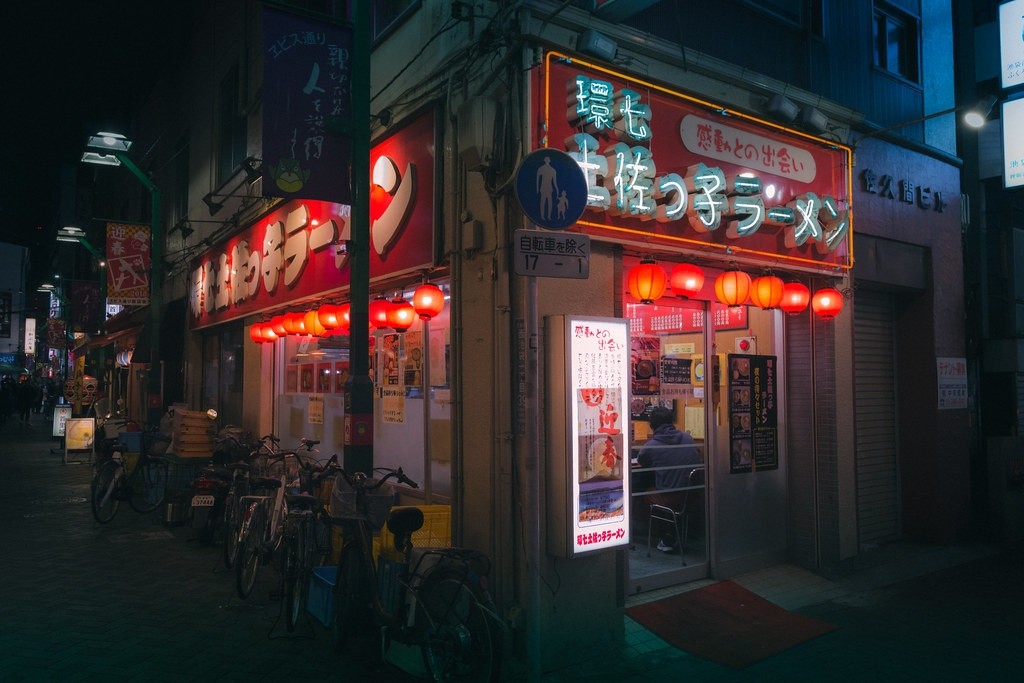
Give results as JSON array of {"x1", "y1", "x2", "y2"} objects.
[
  {"x1": 37, "y1": 282, "x2": 68, "y2": 404},
  {"x1": 80, "y1": 125, "x2": 165, "y2": 360},
  {"x1": 56, "y1": 224, "x2": 107, "y2": 377}
]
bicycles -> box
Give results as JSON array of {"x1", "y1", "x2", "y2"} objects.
[
  {"x1": 313, "y1": 464, "x2": 503, "y2": 683},
  {"x1": 212, "y1": 425, "x2": 280, "y2": 574},
  {"x1": 269, "y1": 451, "x2": 339, "y2": 639},
  {"x1": 90, "y1": 420, "x2": 168, "y2": 525},
  {"x1": 234, "y1": 438, "x2": 320, "y2": 600}
]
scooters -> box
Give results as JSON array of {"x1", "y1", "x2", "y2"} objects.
[{"x1": 188, "y1": 408, "x2": 251, "y2": 548}]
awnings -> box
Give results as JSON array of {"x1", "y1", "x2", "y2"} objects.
[{"x1": 130, "y1": 297, "x2": 187, "y2": 364}]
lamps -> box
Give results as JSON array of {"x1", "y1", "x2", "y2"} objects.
[
  {"x1": 200, "y1": 155, "x2": 264, "y2": 216},
  {"x1": 166, "y1": 215, "x2": 240, "y2": 238},
  {"x1": 81, "y1": 130, "x2": 159, "y2": 193},
  {"x1": 162, "y1": 250, "x2": 195, "y2": 273},
  {"x1": 37, "y1": 284, "x2": 67, "y2": 308},
  {"x1": 855, "y1": 85, "x2": 998, "y2": 152},
  {"x1": 55, "y1": 227, "x2": 107, "y2": 266}
]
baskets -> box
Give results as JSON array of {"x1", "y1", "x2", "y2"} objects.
[{"x1": 330, "y1": 474, "x2": 395, "y2": 522}]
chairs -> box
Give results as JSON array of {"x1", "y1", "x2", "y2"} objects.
[{"x1": 647, "y1": 468, "x2": 704, "y2": 567}]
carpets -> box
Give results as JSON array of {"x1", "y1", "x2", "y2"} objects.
[{"x1": 624, "y1": 580, "x2": 843, "y2": 672}]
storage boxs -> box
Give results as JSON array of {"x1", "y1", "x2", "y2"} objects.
[
  {"x1": 104, "y1": 418, "x2": 143, "y2": 452},
  {"x1": 304, "y1": 503, "x2": 476, "y2": 680}
]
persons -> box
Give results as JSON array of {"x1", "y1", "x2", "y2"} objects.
[
  {"x1": 0, "y1": 373, "x2": 34, "y2": 428},
  {"x1": 636, "y1": 406, "x2": 705, "y2": 552},
  {"x1": 32, "y1": 380, "x2": 63, "y2": 413}
]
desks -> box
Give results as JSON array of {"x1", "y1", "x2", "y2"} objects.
[{"x1": 161, "y1": 454, "x2": 212, "y2": 530}]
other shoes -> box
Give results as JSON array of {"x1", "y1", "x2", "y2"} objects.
[{"x1": 658, "y1": 539, "x2": 672, "y2": 551}]
